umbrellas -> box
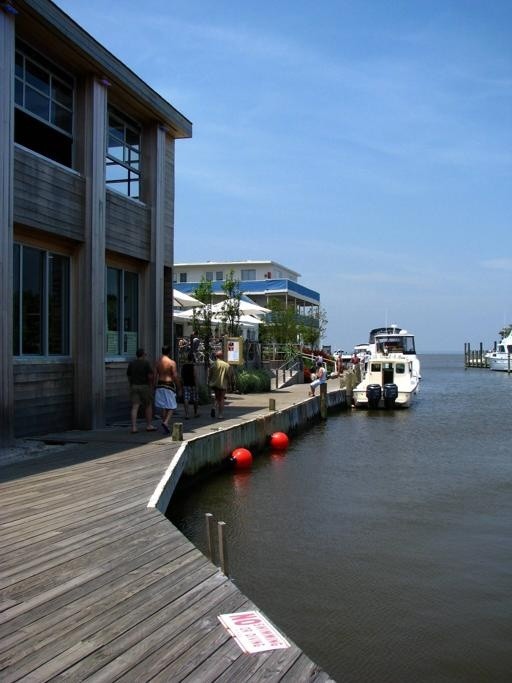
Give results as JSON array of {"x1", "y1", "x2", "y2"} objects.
[
  {"x1": 173, "y1": 288, "x2": 207, "y2": 306},
  {"x1": 171, "y1": 297, "x2": 272, "y2": 330}
]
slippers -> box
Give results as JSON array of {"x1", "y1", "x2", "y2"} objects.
[
  {"x1": 211, "y1": 407, "x2": 217, "y2": 419},
  {"x1": 132, "y1": 422, "x2": 169, "y2": 433}
]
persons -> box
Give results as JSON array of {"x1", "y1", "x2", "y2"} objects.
[
  {"x1": 179, "y1": 353, "x2": 201, "y2": 419},
  {"x1": 177, "y1": 337, "x2": 185, "y2": 353},
  {"x1": 154, "y1": 345, "x2": 184, "y2": 432},
  {"x1": 188, "y1": 330, "x2": 200, "y2": 361},
  {"x1": 180, "y1": 335, "x2": 189, "y2": 353},
  {"x1": 303, "y1": 349, "x2": 360, "y2": 381},
  {"x1": 126, "y1": 347, "x2": 160, "y2": 432},
  {"x1": 204, "y1": 349, "x2": 234, "y2": 419},
  {"x1": 308, "y1": 361, "x2": 327, "y2": 396}
]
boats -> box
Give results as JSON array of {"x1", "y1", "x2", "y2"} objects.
[
  {"x1": 350, "y1": 324, "x2": 423, "y2": 410},
  {"x1": 484, "y1": 331, "x2": 511, "y2": 372}
]
